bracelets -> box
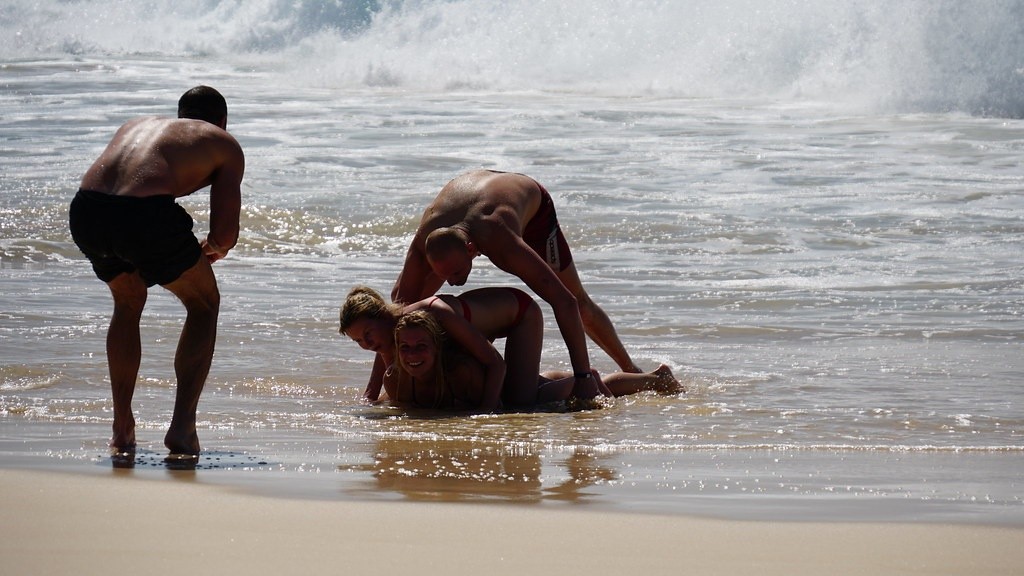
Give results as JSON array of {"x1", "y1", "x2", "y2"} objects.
[{"x1": 573, "y1": 371, "x2": 593, "y2": 378}]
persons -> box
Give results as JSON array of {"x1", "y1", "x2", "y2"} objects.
[
  {"x1": 70, "y1": 86, "x2": 245, "y2": 452},
  {"x1": 339, "y1": 168, "x2": 686, "y2": 416}
]
